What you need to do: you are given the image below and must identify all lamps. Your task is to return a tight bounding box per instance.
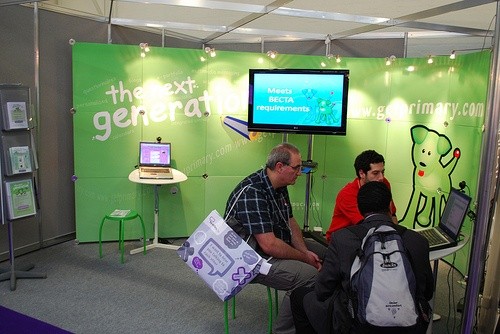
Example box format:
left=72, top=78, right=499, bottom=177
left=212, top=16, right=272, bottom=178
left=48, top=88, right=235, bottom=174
left=327, top=54, right=343, bottom=68
left=384, top=55, right=399, bottom=67
left=448, top=47, right=492, bottom=60
left=427, top=54, right=434, bottom=64
left=199, top=46, right=217, bottom=63
left=138, top=42, right=151, bottom=58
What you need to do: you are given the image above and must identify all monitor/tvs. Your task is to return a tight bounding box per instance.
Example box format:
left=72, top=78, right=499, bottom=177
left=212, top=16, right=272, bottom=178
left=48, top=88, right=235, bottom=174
left=248, top=69, right=349, bottom=135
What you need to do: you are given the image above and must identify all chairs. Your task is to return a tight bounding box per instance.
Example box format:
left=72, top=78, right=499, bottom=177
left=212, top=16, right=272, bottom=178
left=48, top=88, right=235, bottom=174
left=221, top=274, right=279, bottom=334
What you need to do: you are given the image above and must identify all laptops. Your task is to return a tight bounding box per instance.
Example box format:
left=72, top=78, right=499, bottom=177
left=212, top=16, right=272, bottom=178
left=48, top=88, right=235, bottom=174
left=416, top=187, right=472, bottom=251
left=138, top=142, right=172, bottom=179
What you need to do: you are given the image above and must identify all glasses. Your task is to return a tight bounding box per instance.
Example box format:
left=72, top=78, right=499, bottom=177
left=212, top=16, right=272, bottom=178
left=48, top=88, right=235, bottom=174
left=282, top=162, right=303, bottom=171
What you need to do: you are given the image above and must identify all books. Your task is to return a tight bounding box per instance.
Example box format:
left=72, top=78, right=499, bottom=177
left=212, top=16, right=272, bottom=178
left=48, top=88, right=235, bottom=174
left=109, top=209, right=130, bottom=218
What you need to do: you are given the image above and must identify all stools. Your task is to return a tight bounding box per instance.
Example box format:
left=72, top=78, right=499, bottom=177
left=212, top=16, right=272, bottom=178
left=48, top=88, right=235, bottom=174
left=98, top=209, right=148, bottom=265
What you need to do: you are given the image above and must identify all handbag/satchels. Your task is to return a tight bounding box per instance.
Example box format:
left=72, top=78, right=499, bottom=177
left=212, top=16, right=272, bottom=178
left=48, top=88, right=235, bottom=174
left=176, top=185, right=268, bottom=302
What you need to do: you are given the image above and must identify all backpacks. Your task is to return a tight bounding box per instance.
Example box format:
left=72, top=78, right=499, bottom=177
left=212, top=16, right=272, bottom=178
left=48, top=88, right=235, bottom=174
left=347, top=224, right=422, bottom=331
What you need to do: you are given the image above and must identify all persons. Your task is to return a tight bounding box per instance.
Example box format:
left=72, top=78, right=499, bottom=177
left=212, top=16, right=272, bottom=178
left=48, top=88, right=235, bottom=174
left=289, top=181, right=435, bottom=334
left=326, top=150, right=398, bottom=246
left=223, top=142, right=328, bottom=334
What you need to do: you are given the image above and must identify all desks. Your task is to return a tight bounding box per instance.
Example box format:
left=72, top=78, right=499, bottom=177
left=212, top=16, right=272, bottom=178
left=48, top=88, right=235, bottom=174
left=394, top=227, right=470, bottom=323
left=127, top=166, right=188, bottom=259
